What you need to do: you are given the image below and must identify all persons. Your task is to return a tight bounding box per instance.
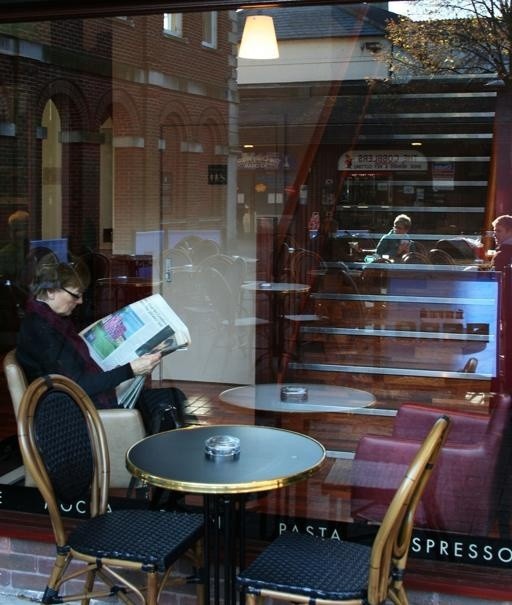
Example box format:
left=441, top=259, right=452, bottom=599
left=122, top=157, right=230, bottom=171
left=1, top=211, right=32, bottom=273
left=15, top=248, right=200, bottom=500
left=490, top=214, right=512, bottom=272
left=377, top=214, right=416, bottom=258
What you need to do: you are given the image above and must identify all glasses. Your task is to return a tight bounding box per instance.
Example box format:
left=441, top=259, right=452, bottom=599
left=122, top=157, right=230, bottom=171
left=60, top=286, right=82, bottom=302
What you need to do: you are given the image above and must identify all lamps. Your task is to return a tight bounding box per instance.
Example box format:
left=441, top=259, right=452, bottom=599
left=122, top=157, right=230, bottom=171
left=236, top=5, right=282, bottom=62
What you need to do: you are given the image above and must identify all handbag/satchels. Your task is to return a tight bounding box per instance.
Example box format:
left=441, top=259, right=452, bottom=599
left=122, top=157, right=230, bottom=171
left=136, top=382, right=186, bottom=435
